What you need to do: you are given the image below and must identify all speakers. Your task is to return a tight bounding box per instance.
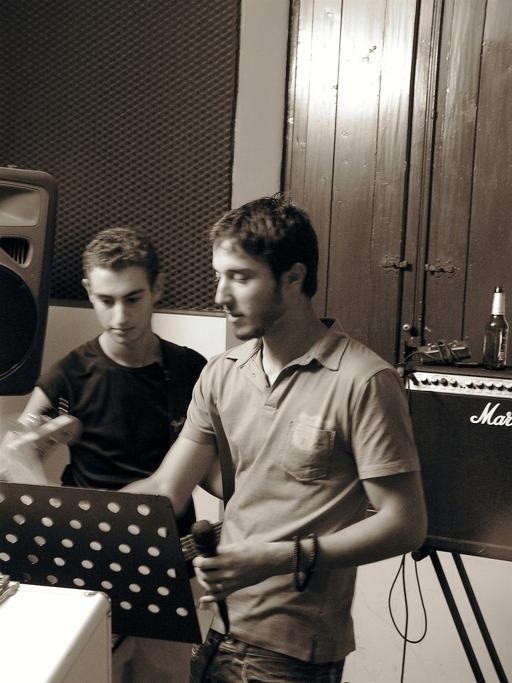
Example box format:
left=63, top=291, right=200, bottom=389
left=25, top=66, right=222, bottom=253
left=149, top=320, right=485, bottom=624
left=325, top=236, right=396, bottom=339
left=403, top=361, right=512, bottom=561
left=0, top=166, right=58, bottom=395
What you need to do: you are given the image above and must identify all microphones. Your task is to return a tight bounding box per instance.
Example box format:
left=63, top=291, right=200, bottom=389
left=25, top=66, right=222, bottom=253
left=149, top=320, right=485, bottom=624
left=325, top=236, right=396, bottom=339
left=193, top=519, right=229, bottom=637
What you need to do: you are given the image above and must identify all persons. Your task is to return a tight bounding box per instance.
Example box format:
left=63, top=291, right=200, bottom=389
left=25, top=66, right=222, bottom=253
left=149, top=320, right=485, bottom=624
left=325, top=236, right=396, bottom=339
left=119, top=199, right=427, bottom=683
left=1, top=228, right=223, bottom=683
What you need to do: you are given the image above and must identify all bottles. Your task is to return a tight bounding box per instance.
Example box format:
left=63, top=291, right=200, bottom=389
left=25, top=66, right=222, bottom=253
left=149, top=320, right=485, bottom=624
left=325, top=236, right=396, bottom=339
left=482, top=284, right=508, bottom=370
